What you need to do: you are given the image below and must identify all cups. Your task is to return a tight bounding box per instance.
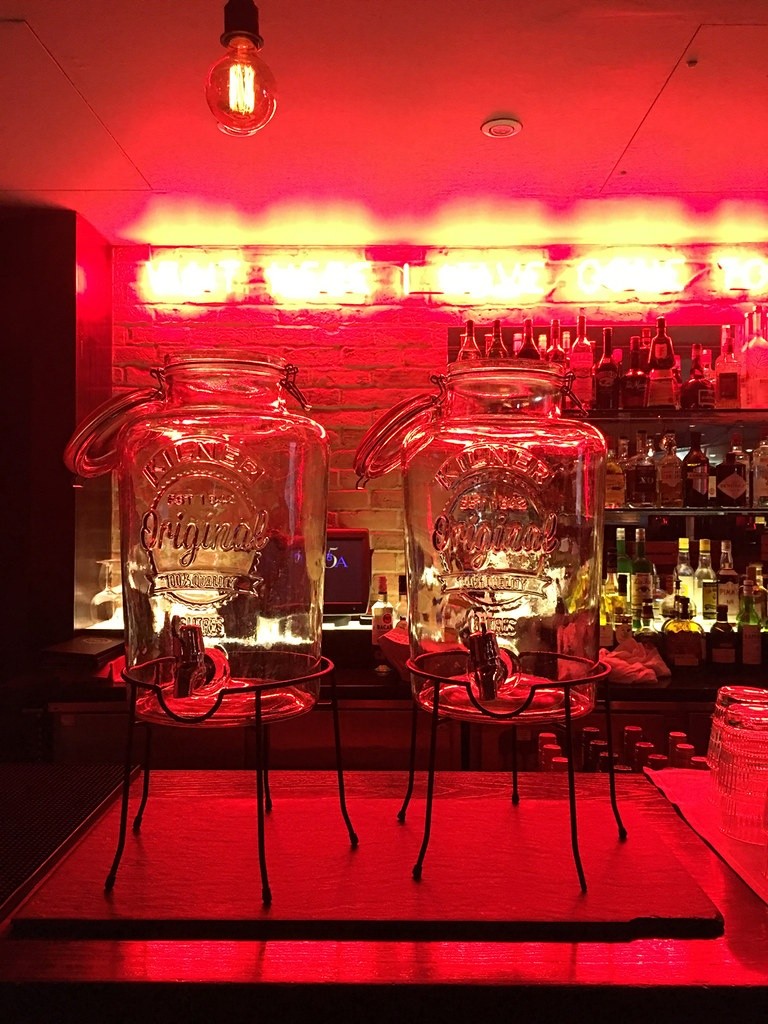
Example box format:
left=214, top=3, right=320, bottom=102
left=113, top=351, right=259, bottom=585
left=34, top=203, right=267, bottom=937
left=708, top=685, right=768, bottom=806
left=719, top=704, right=768, bottom=846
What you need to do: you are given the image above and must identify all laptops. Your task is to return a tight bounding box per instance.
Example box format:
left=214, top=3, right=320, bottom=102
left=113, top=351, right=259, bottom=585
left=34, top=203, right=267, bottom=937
left=262, top=528, right=371, bottom=621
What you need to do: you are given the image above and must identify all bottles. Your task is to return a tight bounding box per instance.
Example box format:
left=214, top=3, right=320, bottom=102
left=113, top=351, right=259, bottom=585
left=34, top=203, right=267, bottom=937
left=401, top=363, right=609, bottom=722
left=108, top=363, right=327, bottom=726
left=372, top=576, right=394, bottom=659
left=464, top=308, right=767, bottom=691
left=393, top=574, right=409, bottom=632
left=536, top=722, right=707, bottom=774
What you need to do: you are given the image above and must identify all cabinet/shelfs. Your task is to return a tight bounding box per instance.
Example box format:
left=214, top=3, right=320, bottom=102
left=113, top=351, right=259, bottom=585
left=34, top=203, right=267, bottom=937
left=559, top=410, right=768, bottom=700
